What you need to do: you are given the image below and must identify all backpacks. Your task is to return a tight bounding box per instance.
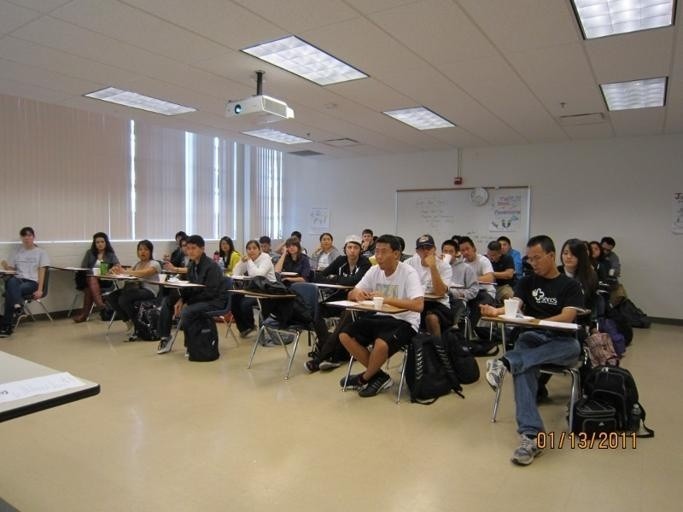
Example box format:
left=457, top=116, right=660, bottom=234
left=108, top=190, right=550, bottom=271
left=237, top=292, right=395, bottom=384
left=132, top=300, right=159, bottom=342
left=580, top=355, right=655, bottom=439
left=407, top=318, right=499, bottom=408
left=583, top=296, right=654, bottom=369
left=245, top=275, right=314, bottom=347
left=183, top=312, right=219, bottom=364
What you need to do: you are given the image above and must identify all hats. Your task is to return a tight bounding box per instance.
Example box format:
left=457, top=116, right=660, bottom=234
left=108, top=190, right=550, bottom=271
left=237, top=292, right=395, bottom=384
left=415, top=234, right=435, bottom=249
left=343, top=235, right=363, bottom=245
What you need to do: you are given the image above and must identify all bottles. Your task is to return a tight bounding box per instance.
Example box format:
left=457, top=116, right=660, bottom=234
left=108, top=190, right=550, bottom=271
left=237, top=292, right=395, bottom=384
left=213, top=250, right=224, bottom=269
left=100, top=261, right=107, bottom=276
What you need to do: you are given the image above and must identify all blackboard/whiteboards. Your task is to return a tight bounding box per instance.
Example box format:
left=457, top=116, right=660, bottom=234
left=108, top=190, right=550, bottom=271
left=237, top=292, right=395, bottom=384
left=396, top=184, right=531, bottom=257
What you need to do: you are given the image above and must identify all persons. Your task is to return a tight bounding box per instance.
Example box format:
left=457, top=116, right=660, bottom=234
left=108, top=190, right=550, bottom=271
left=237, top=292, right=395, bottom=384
left=478, top=234, right=620, bottom=466
left=109, top=230, right=339, bottom=359
left=72, top=231, right=121, bottom=323
left=304, top=229, right=524, bottom=397
left=0, top=226, right=50, bottom=337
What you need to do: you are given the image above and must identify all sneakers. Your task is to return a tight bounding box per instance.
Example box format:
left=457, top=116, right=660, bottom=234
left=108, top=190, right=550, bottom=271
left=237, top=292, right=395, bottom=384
left=484, top=357, right=508, bottom=392
left=240, top=325, right=258, bottom=339
left=0, top=328, right=13, bottom=337
left=304, top=358, right=342, bottom=373
left=15, top=306, right=28, bottom=320
left=510, top=434, right=545, bottom=467
left=358, top=369, right=393, bottom=397
left=156, top=335, right=173, bottom=355
left=340, top=372, right=368, bottom=390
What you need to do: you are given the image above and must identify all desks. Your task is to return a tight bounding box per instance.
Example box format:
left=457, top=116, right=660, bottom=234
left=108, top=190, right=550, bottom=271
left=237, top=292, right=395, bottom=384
left=44, top=263, right=619, bottom=441
left=0, top=349, right=101, bottom=512
left=0, top=269, right=18, bottom=279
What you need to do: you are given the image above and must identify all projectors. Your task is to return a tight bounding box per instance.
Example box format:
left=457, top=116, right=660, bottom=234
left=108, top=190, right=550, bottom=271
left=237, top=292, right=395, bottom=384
left=224, top=93, right=295, bottom=126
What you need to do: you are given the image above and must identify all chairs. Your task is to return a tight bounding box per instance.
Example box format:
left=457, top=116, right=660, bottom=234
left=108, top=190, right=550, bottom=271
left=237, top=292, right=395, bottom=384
left=10, top=267, right=55, bottom=334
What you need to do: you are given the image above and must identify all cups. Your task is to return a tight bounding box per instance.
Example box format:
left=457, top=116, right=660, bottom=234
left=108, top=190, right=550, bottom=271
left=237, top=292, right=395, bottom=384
left=92, top=268, right=100, bottom=276
left=502, top=298, right=519, bottom=318
left=373, top=297, right=384, bottom=309
left=442, top=253, right=452, bottom=264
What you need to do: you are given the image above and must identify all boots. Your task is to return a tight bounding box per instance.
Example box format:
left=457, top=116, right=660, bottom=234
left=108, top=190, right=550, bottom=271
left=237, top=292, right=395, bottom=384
left=72, top=304, right=93, bottom=323
left=92, top=293, right=106, bottom=309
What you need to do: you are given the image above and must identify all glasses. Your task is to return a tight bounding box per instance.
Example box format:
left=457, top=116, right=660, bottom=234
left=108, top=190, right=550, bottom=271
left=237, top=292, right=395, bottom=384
left=526, top=253, right=551, bottom=264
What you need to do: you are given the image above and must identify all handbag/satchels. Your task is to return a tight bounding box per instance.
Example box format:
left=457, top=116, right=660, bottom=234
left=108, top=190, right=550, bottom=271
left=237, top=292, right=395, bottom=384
left=567, top=397, right=619, bottom=441
